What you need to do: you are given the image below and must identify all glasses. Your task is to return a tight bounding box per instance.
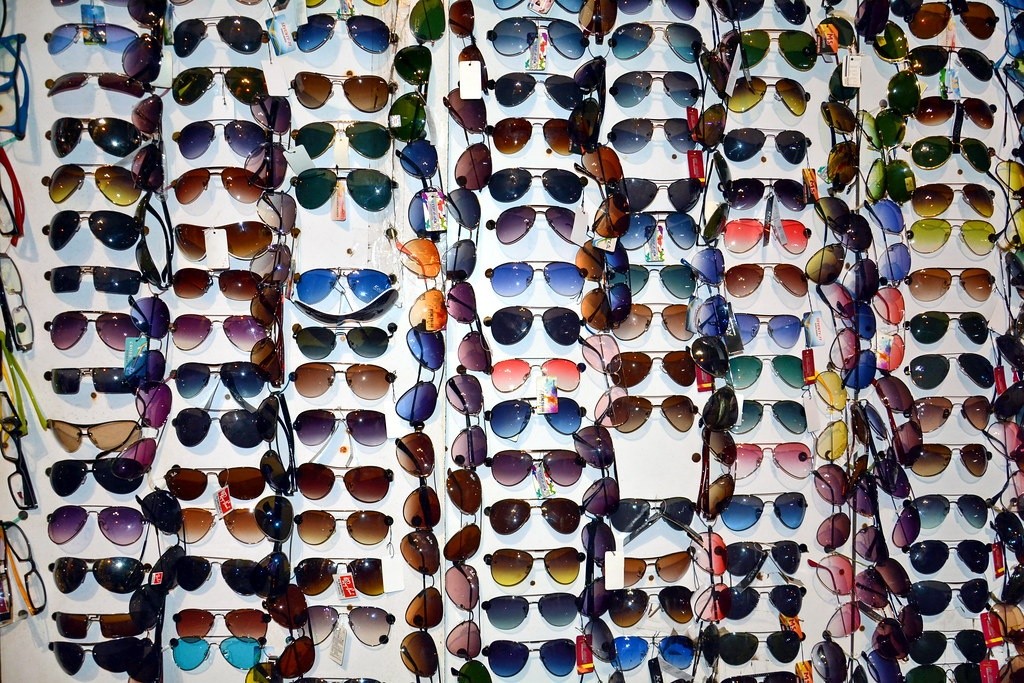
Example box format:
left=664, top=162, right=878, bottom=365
left=0, top=0, right=1024, bottom=683
